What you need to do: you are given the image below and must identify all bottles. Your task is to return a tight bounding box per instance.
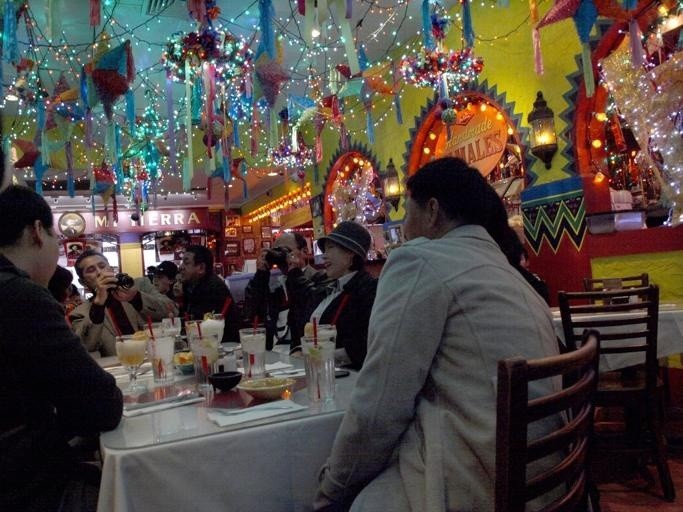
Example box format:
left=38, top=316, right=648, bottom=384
left=596, top=286, right=646, bottom=312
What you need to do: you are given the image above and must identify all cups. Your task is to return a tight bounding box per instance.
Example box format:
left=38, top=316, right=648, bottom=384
left=112, top=316, right=337, bottom=404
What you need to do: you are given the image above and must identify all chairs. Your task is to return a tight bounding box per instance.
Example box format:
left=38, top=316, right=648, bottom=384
left=582, top=273, right=649, bottom=304
left=490, top=328, right=601, bottom=511
left=557, top=284, right=675, bottom=503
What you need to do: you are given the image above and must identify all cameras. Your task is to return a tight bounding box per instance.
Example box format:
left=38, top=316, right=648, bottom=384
left=107, top=273, right=134, bottom=292
left=265, top=246, right=289, bottom=265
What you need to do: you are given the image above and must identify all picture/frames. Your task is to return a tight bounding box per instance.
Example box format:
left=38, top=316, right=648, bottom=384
left=243, top=238, right=256, bottom=254
left=226, top=215, right=240, bottom=228
left=224, top=240, right=240, bottom=256
left=242, top=225, right=253, bottom=234
left=261, top=226, right=272, bottom=239
left=261, top=241, right=271, bottom=249
left=225, top=228, right=237, bottom=237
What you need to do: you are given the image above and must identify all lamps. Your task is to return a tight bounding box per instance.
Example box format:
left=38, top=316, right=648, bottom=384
left=526, top=91, right=557, bottom=170
left=380, top=158, right=401, bottom=211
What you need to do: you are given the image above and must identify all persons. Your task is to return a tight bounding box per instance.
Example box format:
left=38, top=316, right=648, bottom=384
left=147, top=259, right=183, bottom=313
left=68, top=244, right=81, bottom=259
left=521, top=248, right=543, bottom=281
left=214, top=263, right=222, bottom=274
left=160, top=239, right=172, bottom=254
left=48, top=265, right=83, bottom=328
left=0, top=182, right=125, bottom=511
left=70, top=249, right=179, bottom=358
left=180, top=243, right=242, bottom=343
left=309, top=156, right=589, bottom=511
left=242, top=232, right=337, bottom=357
left=287, top=220, right=377, bottom=371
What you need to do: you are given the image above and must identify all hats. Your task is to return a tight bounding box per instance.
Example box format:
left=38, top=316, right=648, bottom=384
left=146, top=259, right=178, bottom=279
left=318, top=219, right=371, bottom=259
